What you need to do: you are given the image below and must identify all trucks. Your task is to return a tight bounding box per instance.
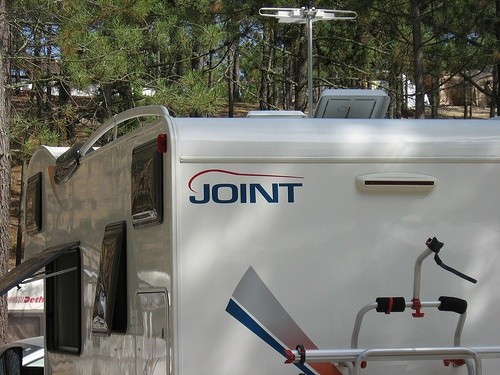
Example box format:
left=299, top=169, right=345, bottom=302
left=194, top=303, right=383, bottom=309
left=0, top=88, right=499, bottom=375
